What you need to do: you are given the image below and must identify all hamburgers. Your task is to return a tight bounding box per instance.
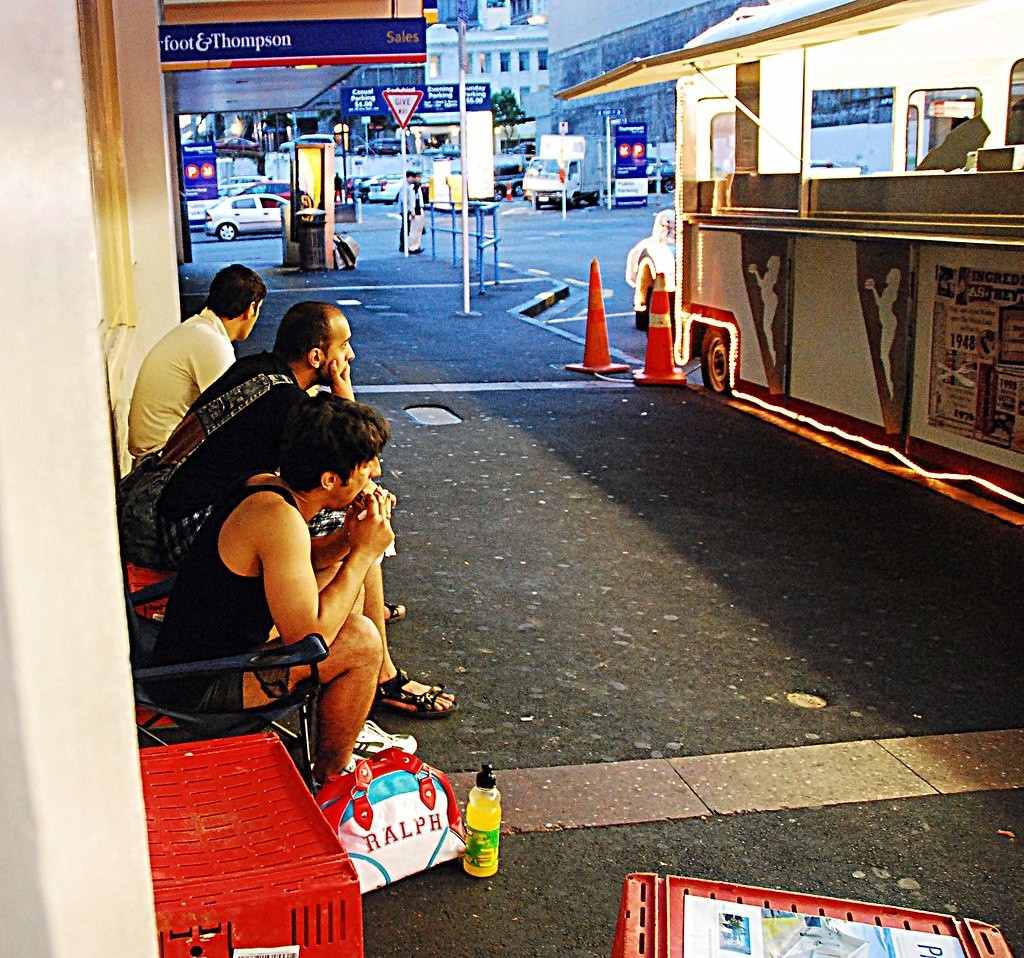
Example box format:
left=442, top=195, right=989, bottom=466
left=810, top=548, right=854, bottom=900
left=352, top=480, right=378, bottom=509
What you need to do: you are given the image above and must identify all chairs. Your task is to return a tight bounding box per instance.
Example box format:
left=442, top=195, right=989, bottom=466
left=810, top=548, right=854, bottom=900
left=125, top=565, right=327, bottom=786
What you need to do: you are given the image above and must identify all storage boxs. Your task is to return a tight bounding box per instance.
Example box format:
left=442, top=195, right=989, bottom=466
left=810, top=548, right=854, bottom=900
left=611, top=874, right=1014, bottom=958
left=138, top=730, right=363, bottom=958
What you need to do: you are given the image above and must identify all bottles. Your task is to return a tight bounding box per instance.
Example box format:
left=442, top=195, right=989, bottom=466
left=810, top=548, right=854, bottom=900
left=462, top=762, right=501, bottom=877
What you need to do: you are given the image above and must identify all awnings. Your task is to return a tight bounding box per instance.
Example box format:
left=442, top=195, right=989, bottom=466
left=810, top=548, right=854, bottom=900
left=162, top=65, right=361, bottom=113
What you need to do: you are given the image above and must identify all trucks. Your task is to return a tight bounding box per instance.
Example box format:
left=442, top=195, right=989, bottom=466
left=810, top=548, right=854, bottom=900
left=522, top=134, right=616, bottom=208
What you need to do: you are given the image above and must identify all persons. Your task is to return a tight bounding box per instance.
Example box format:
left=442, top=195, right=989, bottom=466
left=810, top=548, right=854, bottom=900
left=153, top=302, right=455, bottom=712
left=334, top=172, right=356, bottom=203
left=146, top=392, right=393, bottom=798
left=420, top=135, right=451, bottom=161
left=398, top=171, right=425, bottom=254
left=127, top=264, right=267, bottom=472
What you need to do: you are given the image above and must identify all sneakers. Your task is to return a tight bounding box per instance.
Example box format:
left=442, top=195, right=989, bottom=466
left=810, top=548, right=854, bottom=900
left=310, top=753, right=368, bottom=797
left=352, top=720, right=418, bottom=758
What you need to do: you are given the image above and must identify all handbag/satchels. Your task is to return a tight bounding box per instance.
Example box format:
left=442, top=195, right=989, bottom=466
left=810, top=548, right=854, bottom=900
left=316, top=747, right=467, bottom=895
left=118, top=454, right=170, bottom=567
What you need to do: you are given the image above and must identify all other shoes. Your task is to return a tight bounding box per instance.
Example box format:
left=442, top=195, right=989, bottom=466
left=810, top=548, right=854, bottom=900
left=409, top=247, right=425, bottom=255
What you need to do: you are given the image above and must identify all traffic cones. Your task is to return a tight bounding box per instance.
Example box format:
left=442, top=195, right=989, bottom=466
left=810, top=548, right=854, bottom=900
left=505, top=180, right=515, bottom=202
left=632, top=272, right=689, bottom=386
left=566, top=256, right=631, bottom=372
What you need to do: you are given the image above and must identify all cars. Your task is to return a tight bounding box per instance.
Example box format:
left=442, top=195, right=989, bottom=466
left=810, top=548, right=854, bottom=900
left=646, top=156, right=676, bottom=194
left=501, top=140, right=537, bottom=155
left=355, top=173, right=429, bottom=207
left=204, top=194, right=291, bottom=240
left=215, top=137, right=263, bottom=157
left=234, top=180, right=291, bottom=207
left=420, top=142, right=462, bottom=159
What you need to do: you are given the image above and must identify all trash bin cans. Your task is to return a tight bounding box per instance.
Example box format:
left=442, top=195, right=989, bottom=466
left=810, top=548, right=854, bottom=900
left=296, top=207, right=327, bottom=271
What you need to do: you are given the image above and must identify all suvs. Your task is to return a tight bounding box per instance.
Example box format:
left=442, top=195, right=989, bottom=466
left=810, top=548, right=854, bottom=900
left=217, top=175, right=269, bottom=198
left=278, top=133, right=345, bottom=156
left=354, top=137, right=411, bottom=156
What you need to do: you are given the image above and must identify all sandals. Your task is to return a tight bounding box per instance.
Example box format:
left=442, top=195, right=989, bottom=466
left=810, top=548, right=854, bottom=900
left=375, top=668, right=460, bottom=718
left=384, top=602, right=406, bottom=622
